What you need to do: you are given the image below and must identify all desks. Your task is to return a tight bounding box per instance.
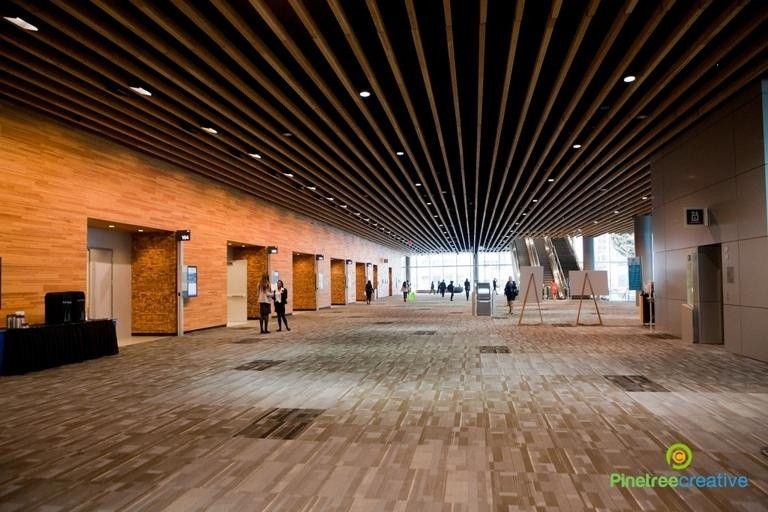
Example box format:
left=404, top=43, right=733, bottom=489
left=0, top=320, right=119, bottom=376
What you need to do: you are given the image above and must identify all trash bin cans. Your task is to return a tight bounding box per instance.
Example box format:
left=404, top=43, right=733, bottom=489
left=476, top=282, right=496, bottom=317
left=639, top=293, right=656, bottom=326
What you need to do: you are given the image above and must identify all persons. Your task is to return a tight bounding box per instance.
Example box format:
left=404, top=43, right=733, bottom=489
left=271, top=279, right=290, bottom=332
left=492, top=278, right=499, bottom=295
left=438, top=279, right=447, bottom=297
left=550, top=279, right=557, bottom=300
left=255, top=272, right=276, bottom=333
left=365, top=281, right=373, bottom=304
left=437, top=281, right=441, bottom=294
left=448, top=281, right=455, bottom=301
left=428, top=281, right=436, bottom=295
left=464, top=278, right=471, bottom=300
left=401, top=281, right=409, bottom=301
left=504, top=276, right=518, bottom=313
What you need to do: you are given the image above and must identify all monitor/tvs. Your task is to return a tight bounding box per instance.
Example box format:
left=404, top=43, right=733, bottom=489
left=478, top=288, right=490, bottom=294
